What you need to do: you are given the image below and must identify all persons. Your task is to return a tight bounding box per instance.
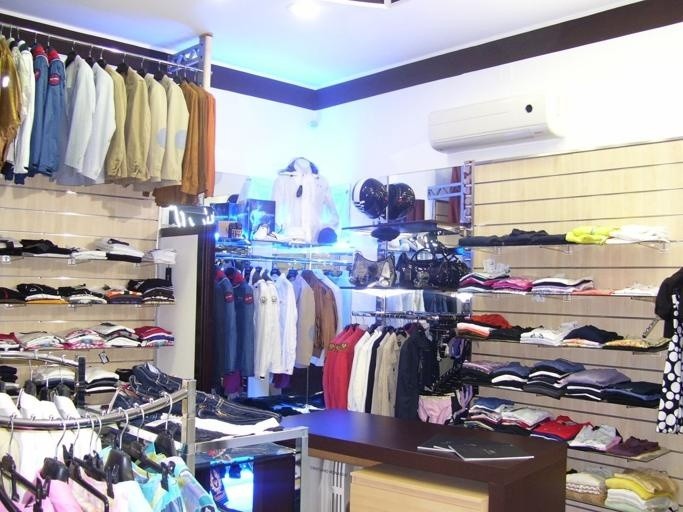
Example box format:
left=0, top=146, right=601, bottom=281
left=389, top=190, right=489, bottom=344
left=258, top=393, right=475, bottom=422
left=248, top=201, right=274, bottom=240
left=272, top=157, right=338, bottom=243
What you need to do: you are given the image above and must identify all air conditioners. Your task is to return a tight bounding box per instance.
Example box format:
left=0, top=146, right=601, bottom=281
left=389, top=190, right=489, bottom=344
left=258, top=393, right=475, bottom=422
left=427, top=92, right=566, bottom=154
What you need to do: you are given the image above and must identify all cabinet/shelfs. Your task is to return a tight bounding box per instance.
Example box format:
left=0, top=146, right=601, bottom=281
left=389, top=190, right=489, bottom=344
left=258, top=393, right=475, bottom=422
left=457, top=238, right=672, bottom=511
left=339, top=220, right=461, bottom=292
left=273, top=409, right=569, bottom=511
left=0, top=252, right=175, bottom=350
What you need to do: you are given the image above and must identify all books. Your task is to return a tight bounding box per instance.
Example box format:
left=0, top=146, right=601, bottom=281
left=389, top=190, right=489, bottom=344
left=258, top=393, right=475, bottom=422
left=416, top=438, right=533, bottom=463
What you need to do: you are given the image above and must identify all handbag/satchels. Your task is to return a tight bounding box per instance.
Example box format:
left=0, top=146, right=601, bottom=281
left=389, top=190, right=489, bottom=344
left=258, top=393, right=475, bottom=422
left=349, top=247, right=471, bottom=289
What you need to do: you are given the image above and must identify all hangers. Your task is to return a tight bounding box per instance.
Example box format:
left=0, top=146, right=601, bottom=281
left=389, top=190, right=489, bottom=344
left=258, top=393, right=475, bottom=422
left=0, top=393, right=177, bottom=512
left=345, top=312, right=420, bottom=337
left=1, top=24, right=198, bottom=85
left=217, top=252, right=324, bottom=281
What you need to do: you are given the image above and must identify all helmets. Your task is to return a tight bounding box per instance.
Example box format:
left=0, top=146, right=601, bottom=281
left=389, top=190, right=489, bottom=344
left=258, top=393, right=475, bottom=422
left=388, top=183, right=415, bottom=220
left=352, top=178, right=387, bottom=218
